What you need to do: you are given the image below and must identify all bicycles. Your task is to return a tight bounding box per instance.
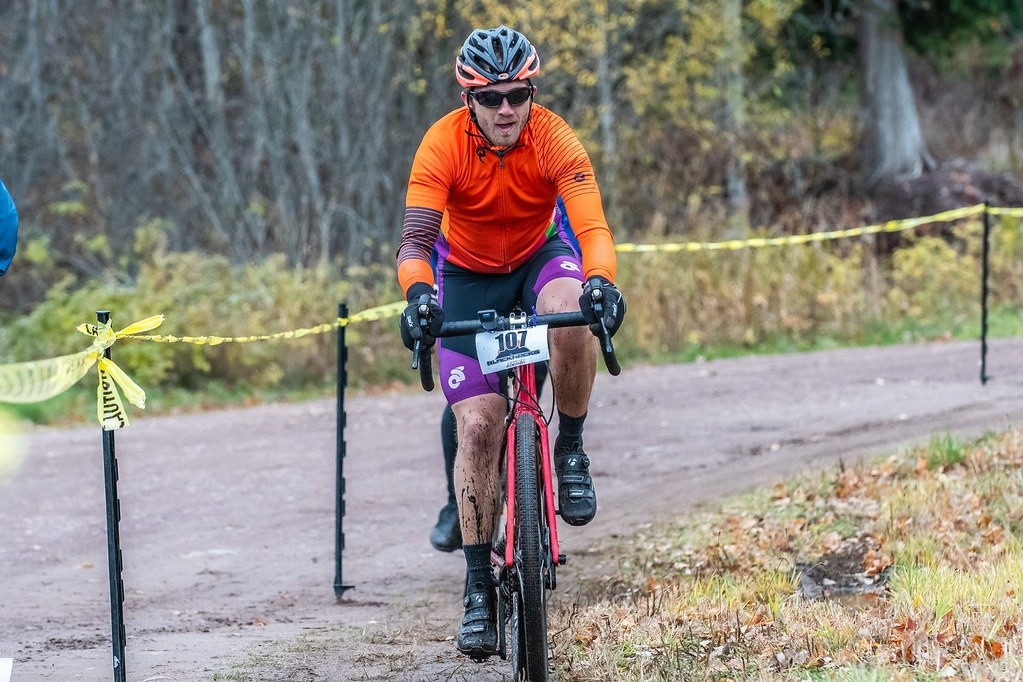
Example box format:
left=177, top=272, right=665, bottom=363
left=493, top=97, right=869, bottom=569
left=411, top=278, right=621, bottom=681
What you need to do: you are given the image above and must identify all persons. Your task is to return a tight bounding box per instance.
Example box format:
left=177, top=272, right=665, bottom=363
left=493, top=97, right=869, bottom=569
left=394, top=25, right=626, bottom=654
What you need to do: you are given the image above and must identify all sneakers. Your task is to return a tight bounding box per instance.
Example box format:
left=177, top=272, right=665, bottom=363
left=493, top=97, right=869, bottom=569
left=456, top=572, right=499, bottom=657
left=430, top=496, right=462, bottom=552
left=552, top=436, right=598, bottom=526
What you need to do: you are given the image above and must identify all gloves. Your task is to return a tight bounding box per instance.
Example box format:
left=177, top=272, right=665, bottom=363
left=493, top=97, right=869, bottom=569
left=580, top=275, right=629, bottom=339
left=397, top=293, right=446, bottom=354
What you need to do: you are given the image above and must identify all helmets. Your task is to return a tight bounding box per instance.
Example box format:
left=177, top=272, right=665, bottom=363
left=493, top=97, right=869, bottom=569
left=455, top=24, right=540, bottom=87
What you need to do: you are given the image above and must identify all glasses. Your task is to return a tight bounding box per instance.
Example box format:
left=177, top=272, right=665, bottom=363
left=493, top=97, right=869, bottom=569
left=469, top=86, right=534, bottom=107
